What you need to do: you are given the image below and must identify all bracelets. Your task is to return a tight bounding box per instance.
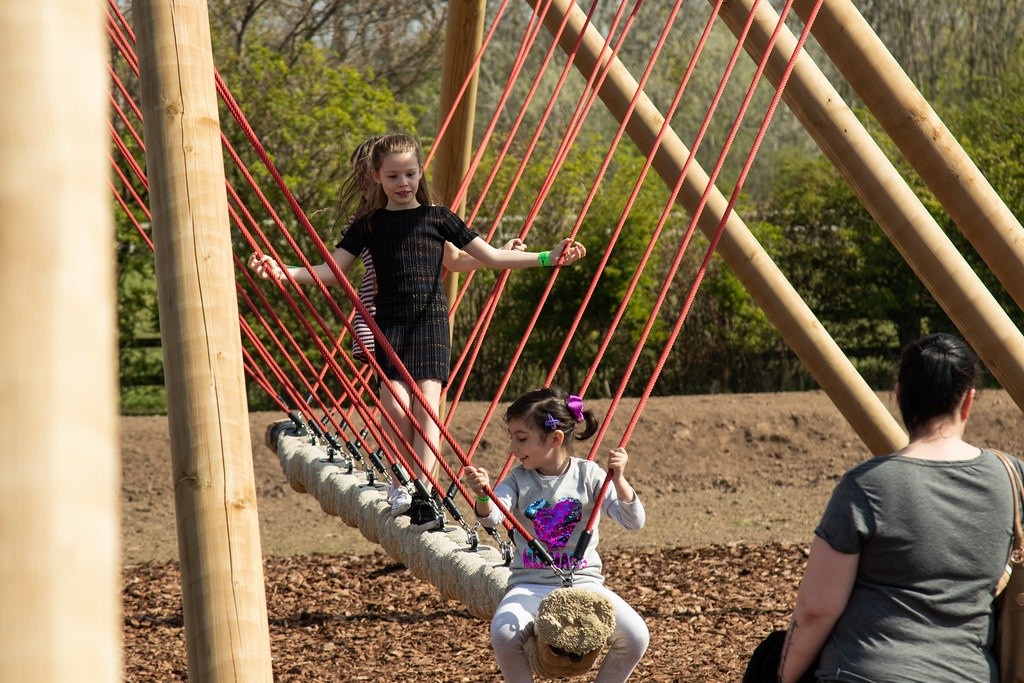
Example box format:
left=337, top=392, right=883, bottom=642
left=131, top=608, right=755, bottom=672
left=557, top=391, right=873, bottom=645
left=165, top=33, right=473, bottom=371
left=539, top=251, right=551, bottom=266
left=477, top=496, right=489, bottom=502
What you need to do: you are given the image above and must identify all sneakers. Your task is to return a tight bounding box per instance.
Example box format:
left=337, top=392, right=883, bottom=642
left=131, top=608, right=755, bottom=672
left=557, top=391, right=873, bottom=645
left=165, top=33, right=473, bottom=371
left=387, top=484, right=413, bottom=516
left=409, top=490, right=442, bottom=532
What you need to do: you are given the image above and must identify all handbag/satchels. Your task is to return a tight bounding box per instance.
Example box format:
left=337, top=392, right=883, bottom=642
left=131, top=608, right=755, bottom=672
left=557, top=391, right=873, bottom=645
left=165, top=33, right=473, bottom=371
left=987, top=446, right=1024, bottom=683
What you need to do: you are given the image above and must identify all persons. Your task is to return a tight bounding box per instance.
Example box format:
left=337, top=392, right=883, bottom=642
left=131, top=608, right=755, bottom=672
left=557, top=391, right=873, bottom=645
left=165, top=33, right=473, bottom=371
left=263, top=136, right=527, bottom=516
left=249, top=134, right=587, bottom=531
left=465, top=389, right=649, bottom=683
left=778, top=334, right=1024, bottom=683
